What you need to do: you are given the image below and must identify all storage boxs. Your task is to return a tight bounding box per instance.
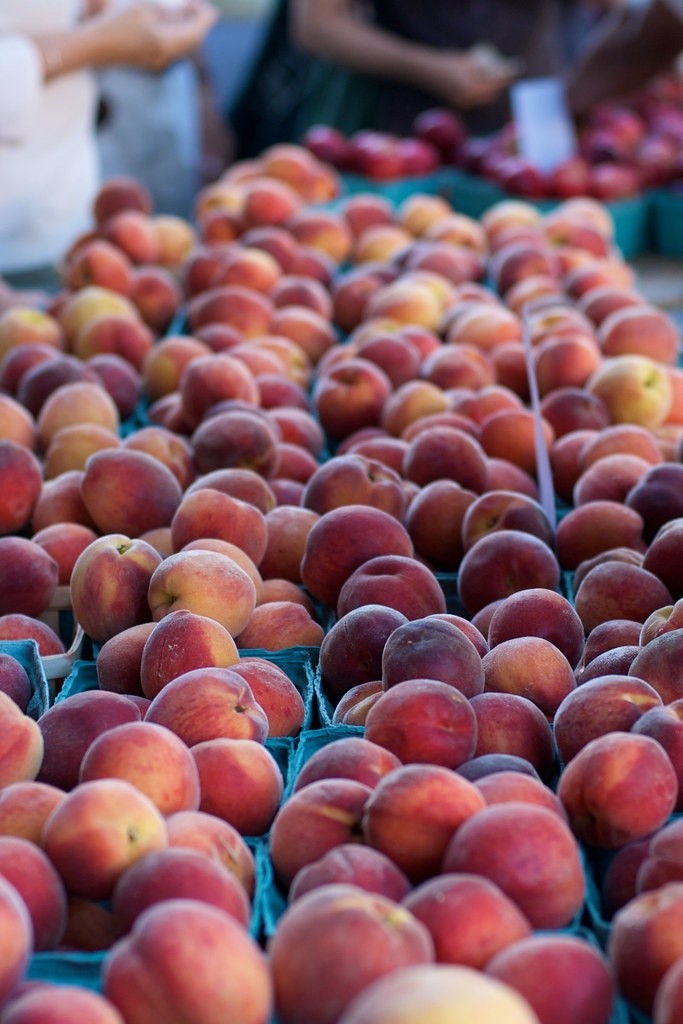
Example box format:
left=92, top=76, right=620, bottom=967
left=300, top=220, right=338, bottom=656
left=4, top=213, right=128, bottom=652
left=0, top=168, right=683, bottom=1024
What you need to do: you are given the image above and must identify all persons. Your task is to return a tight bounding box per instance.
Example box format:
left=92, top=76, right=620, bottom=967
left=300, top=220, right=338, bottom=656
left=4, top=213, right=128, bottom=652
left=228, top=0, right=543, bottom=163
left=0, top=0, right=219, bottom=291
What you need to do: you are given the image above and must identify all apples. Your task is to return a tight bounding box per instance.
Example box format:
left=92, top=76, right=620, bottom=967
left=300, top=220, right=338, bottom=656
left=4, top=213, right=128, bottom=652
left=301, top=70, right=682, bottom=197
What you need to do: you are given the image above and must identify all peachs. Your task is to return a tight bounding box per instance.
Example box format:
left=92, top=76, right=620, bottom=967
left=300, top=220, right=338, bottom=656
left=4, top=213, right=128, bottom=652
left=0, top=142, right=683, bottom=1024
